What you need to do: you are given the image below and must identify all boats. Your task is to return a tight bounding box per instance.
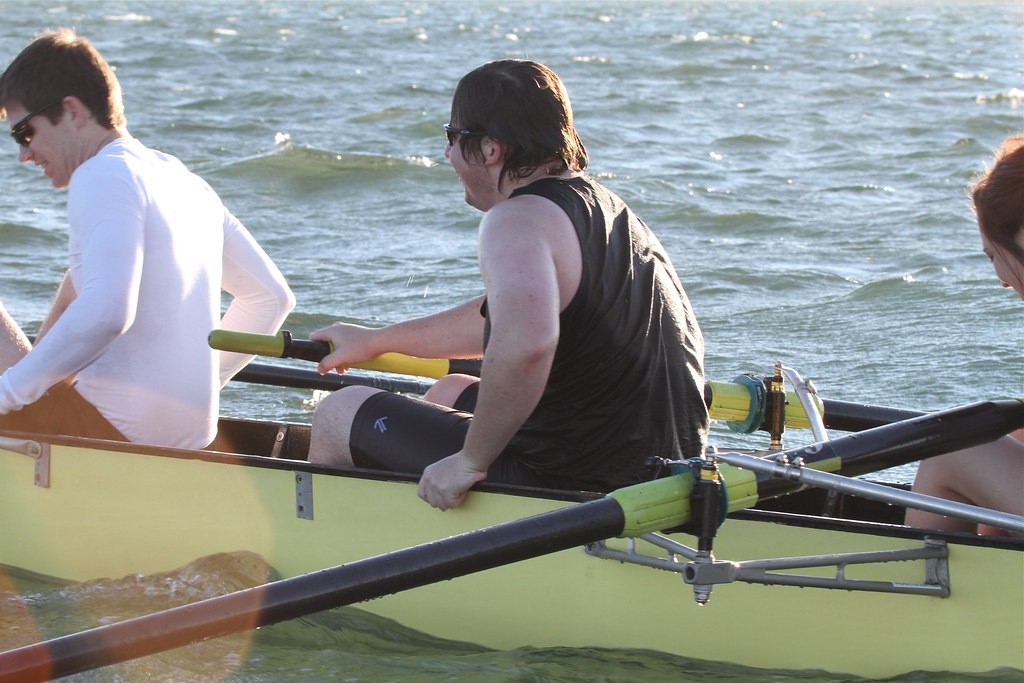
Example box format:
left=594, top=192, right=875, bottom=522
left=0, top=416, right=1023, bottom=683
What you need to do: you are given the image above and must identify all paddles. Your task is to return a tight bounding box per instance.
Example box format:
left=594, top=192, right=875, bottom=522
left=0, top=398, right=1022, bottom=682
left=206, top=327, right=923, bottom=430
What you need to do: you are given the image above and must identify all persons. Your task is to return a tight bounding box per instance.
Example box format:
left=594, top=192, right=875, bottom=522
left=0, top=27, right=298, bottom=451
left=903, top=144, right=1024, bottom=539
left=306, top=59, right=711, bottom=512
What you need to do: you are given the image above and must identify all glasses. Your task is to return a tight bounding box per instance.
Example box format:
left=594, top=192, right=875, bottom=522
left=443, top=123, right=486, bottom=146
left=9, top=91, right=89, bottom=148
